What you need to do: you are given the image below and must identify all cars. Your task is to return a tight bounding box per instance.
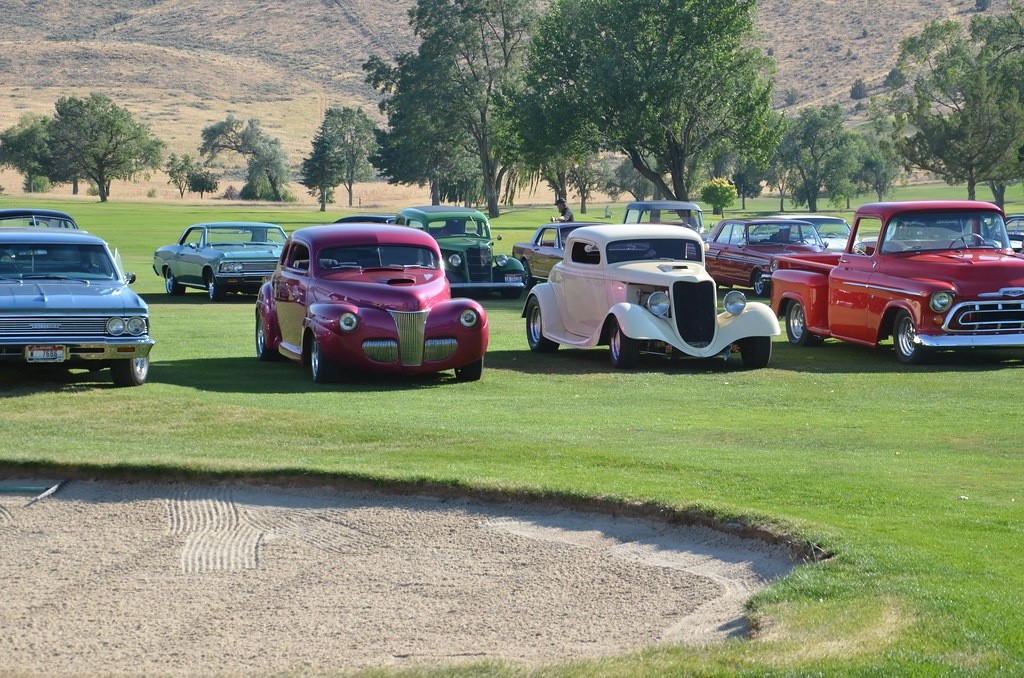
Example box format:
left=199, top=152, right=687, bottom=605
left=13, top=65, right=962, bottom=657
left=255, top=223, right=488, bottom=383
left=0, top=227, right=156, bottom=385
left=0, top=201, right=1024, bottom=302
left=521, top=224, right=782, bottom=369
left=770, top=201, right=1024, bottom=363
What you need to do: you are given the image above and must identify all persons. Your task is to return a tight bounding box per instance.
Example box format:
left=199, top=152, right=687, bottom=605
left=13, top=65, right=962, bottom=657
left=550, top=197, right=574, bottom=222
left=605, top=205, right=612, bottom=219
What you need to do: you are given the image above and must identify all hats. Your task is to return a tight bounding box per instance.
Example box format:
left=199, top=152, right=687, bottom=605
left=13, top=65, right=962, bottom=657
left=554, top=198, right=565, bottom=205
